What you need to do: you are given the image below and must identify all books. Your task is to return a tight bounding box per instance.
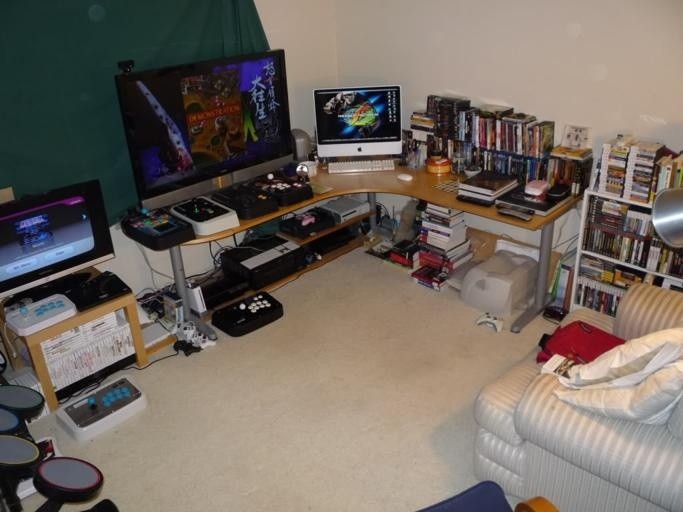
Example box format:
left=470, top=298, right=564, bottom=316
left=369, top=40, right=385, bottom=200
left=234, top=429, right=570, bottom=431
left=362, top=201, right=477, bottom=293
left=404, top=93, right=594, bottom=223
left=548, top=133, right=682, bottom=317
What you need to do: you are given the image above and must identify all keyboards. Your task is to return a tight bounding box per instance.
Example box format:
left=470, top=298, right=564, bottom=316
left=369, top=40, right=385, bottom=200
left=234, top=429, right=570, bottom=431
left=327, top=160, right=395, bottom=174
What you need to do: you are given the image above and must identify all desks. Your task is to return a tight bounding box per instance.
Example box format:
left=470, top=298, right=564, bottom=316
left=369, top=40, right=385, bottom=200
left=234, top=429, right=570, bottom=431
left=0, top=259, right=150, bottom=412
left=168, top=156, right=585, bottom=337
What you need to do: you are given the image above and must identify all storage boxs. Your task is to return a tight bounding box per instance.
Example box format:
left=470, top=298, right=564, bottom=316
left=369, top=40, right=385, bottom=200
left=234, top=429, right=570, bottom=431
left=455, top=248, right=540, bottom=319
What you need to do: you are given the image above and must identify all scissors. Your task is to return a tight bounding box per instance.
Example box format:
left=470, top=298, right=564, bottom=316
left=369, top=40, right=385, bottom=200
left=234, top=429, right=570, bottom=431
left=411, top=139, right=421, bottom=163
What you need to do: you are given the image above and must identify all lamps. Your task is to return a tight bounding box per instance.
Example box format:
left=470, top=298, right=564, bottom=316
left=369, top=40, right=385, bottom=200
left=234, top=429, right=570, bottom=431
left=648, top=186, right=682, bottom=250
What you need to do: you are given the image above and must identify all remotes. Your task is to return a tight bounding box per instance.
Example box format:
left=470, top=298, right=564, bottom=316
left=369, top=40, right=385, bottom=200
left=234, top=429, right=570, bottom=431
left=456, top=195, right=492, bottom=207
left=497, top=208, right=532, bottom=222
left=495, top=201, right=535, bottom=215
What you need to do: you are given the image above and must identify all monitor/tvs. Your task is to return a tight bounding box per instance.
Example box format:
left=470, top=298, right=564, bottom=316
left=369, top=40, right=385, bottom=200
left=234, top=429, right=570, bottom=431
left=0, top=178, right=115, bottom=310
left=114, top=49, right=294, bottom=214
left=312, top=85, right=402, bottom=157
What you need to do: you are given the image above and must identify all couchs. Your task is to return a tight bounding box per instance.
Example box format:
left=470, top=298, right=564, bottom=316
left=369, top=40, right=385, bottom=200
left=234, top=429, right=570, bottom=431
left=467, top=279, right=682, bottom=512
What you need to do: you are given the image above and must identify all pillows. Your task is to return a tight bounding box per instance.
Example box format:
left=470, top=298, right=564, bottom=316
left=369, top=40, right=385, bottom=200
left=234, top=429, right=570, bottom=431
left=556, top=326, right=681, bottom=390
left=551, top=360, right=682, bottom=425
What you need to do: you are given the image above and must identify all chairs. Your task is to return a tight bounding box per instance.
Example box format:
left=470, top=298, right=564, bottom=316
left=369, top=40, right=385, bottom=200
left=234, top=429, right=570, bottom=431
left=409, top=477, right=563, bottom=511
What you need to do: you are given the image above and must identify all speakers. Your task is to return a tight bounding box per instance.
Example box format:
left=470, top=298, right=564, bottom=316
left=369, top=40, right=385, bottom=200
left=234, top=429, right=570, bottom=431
left=290, top=129, right=312, bottom=162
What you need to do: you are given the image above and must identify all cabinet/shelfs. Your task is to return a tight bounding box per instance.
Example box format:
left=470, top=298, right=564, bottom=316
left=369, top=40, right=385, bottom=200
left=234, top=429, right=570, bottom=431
left=564, top=184, right=683, bottom=319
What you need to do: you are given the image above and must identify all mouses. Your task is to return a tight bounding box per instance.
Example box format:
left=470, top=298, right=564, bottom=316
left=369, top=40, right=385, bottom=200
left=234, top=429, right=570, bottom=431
left=396, top=172, right=412, bottom=181
